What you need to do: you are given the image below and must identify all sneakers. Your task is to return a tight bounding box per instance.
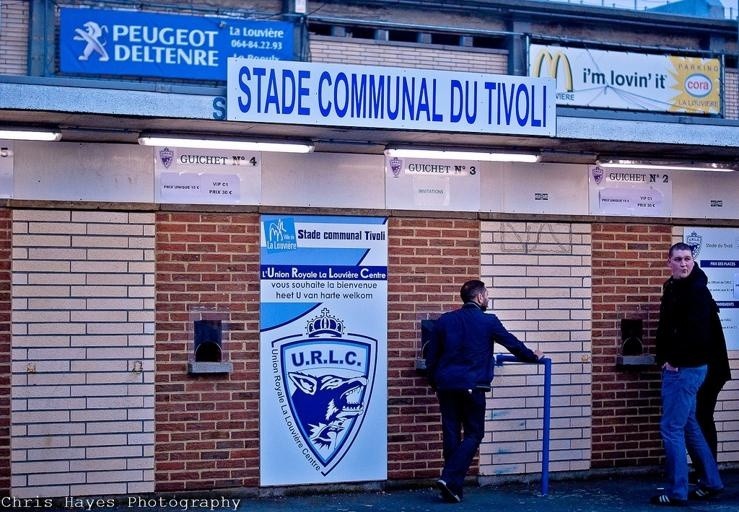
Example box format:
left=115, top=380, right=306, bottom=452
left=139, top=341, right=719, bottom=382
left=649, top=483, right=724, bottom=506
left=436, top=480, right=460, bottom=502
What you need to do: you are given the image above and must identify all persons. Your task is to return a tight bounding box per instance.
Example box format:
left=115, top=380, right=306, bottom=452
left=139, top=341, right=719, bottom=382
left=688, top=286, right=731, bottom=485
left=427, top=280, right=545, bottom=503
left=651, top=243, right=724, bottom=507
